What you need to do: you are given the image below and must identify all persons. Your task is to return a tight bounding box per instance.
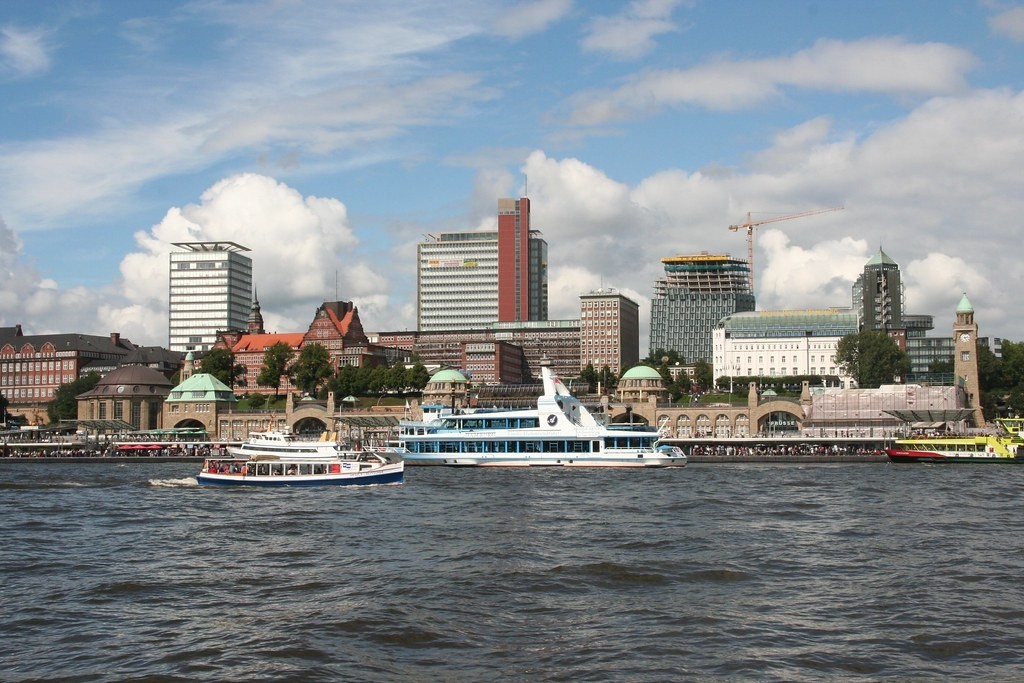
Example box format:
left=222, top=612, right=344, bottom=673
left=693, top=390, right=710, bottom=404
left=1, top=426, right=328, bottom=474
left=659, top=416, right=1022, bottom=457
left=453, top=403, right=533, bottom=430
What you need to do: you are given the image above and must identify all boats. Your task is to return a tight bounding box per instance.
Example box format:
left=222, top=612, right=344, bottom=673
left=225, top=431, right=338, bottom=458
left=386, top=364, right=687, bottom=469
left=883, top=418, right=1024, bottom=463
left=196, top=450, right=405, bottom=488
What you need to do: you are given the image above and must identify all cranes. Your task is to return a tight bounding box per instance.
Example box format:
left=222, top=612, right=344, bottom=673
left=728, top=205, right=845, bottom=299
